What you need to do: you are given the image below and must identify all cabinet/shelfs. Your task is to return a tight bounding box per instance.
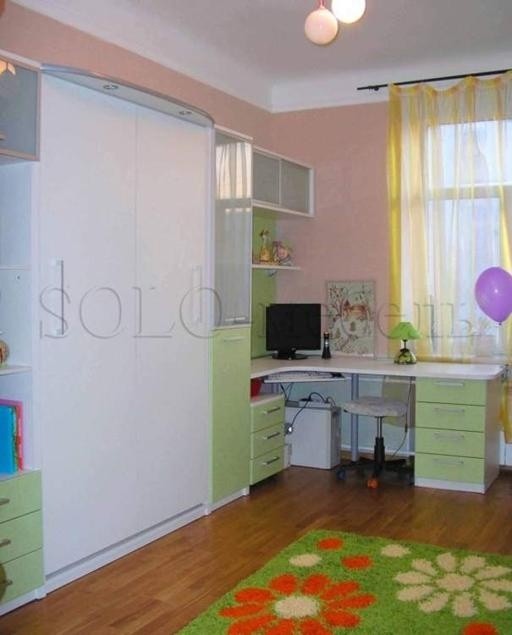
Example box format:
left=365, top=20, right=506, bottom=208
left=0, top=466, right=45, bottom=605
left=212, top=124, right=253, bottom=331
left=0, top=48, right=211, bottom=602
left=211, top=323, right=252, bottom=504
left=250, top=392, right=285, bottom=486
left=414, top=377, right=501, bottom=495
left=252, top=144, right=314, bottom=218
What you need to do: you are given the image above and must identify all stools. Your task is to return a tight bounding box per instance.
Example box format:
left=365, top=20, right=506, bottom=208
left=336, top=396, right=408, bottom=489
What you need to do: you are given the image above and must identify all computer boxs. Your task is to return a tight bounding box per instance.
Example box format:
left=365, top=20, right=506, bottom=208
left=284, top=400, right=342, bottom=470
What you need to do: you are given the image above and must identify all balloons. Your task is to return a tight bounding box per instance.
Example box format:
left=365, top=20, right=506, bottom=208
left=473, top=266, right=512, bottom=325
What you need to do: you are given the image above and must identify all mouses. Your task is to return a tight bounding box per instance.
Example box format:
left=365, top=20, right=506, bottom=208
left=332, top=373, right=341, bottom=377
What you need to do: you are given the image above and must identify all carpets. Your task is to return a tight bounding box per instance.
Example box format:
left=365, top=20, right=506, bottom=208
left=171, top=528, right=512, bottom=635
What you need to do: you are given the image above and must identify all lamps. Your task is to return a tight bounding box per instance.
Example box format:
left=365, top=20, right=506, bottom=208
left=387, top=321, right=421, bottom=365
left=303, top=0, right=338, bottom=46
left=331, top=0, right=366, bottom=25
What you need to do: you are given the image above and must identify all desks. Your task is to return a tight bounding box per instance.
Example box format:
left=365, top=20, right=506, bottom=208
left=251, top=353, right=507, bottom=464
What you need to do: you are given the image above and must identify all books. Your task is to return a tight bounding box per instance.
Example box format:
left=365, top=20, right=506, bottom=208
left=0, top=398, right=25, bottom=474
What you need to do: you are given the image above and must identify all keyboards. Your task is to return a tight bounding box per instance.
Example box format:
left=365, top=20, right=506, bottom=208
left=268, top=371, right=335, bottom=380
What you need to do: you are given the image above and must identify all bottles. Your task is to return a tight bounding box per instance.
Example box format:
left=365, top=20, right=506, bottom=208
left=322, top=331, right=331, bottom=358
left=259, top=230, right=271, bottom=263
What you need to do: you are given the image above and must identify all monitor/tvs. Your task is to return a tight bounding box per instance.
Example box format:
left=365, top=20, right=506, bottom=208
left=268, top=303, right=321, bottom=360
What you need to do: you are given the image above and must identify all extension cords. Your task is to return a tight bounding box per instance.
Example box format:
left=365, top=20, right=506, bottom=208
left=299, top=398, right=332, bottom=408
